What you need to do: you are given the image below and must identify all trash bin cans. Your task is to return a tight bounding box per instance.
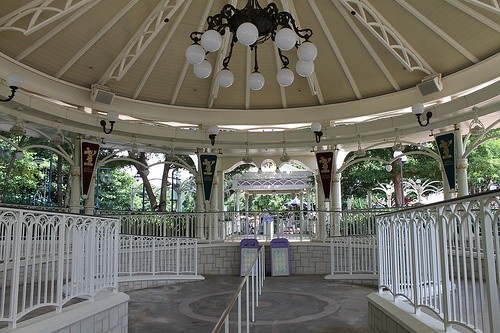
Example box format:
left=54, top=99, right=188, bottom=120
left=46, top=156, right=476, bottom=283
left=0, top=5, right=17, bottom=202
left=269, top=237, right=291, bottom=277
left=239, top=238, right=259, bottom=277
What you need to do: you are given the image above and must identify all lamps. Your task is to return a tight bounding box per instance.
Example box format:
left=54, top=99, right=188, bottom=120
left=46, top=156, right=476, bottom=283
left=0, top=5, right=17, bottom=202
left=311, top=121, right=323, bottom=143
left=207, top=125, right=219, bottom=146
left=100, top=110, right=119, bottom=134
left=0, top=72, right=24, bottom=102
left=411, top=102, right=432, bottom=126
left=185, top=0, right=318, bottom=91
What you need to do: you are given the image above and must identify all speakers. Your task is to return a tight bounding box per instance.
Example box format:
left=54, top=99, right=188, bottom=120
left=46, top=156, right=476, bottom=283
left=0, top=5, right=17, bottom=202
left=90, top=89, right=115, bottom=105
left=417, top=77, right=443, bottom=96
